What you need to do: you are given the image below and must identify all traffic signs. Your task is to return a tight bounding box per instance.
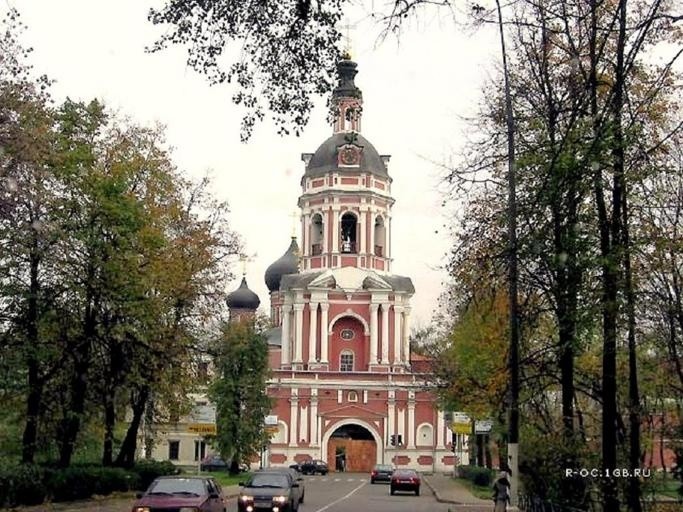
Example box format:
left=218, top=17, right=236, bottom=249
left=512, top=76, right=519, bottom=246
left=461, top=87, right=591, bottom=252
left=187, top=424, right=216, bottom=433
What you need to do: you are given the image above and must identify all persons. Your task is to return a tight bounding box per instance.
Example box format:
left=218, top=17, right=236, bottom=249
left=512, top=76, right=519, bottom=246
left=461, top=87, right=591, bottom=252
left=492, top=471, right=510, bottom=512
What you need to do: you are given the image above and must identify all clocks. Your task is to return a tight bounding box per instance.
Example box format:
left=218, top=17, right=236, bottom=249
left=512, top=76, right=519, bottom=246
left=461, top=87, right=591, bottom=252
left=340, top=149, right=358, bottom=166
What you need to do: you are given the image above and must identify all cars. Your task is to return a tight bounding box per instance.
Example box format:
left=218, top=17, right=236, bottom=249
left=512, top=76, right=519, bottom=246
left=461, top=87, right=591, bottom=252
left=132, top=476, right=227, bottom=512
left=371, top=464, right=396, bottom=484
left=237, top=466, right=305, bottom=512
left=201, top=454, right=250, bottom=472
left=391, top=468, right=420, bottom=496
left=289, top=459, right=329, bottom=475
